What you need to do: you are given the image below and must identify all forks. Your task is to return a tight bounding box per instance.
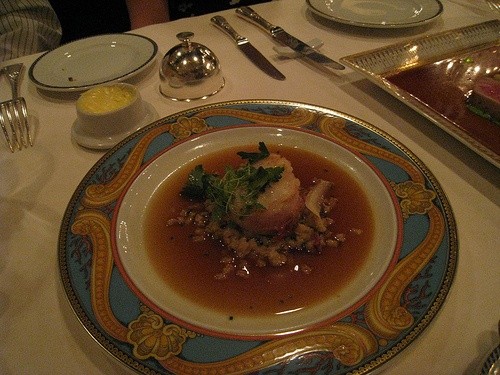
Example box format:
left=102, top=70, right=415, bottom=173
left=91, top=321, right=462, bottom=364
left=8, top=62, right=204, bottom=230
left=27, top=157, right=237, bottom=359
left=486, top=0, right=500, bottom=11
left=0, top=63, right=32, bottom=153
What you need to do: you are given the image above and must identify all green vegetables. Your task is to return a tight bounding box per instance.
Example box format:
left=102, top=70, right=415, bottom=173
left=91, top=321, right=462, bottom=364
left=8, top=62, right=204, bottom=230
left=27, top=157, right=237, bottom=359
left=466, top=102, right=500, bottom=129
left=177, top=141, right=319, bottom=255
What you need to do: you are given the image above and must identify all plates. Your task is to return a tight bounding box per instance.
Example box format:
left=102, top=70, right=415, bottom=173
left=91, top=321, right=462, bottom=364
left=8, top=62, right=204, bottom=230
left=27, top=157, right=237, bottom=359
left=305, top=0, right=444, bottom=29
left=28, top=32, right=159, bottom=92
left=57, top=98, right=459, bottom=375
left=338, top=20, right=500, bottom=168
left=476, top=342, right=500, bottom=375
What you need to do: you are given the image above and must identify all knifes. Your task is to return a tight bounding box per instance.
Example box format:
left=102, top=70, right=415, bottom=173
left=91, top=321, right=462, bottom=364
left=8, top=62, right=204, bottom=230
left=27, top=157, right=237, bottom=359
left=210, top=15, right=287, bottom=81
left=235, top=6, right=346, bottom=70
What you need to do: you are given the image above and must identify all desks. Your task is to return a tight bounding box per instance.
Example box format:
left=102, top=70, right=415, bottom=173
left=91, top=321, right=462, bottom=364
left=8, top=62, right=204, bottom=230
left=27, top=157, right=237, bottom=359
left=0, top=0, right=500, bottom=375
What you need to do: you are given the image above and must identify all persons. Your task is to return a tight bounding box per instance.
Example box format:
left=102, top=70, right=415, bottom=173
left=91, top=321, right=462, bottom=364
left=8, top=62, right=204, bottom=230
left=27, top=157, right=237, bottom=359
left=0, top=0, right=278, bottom=63
